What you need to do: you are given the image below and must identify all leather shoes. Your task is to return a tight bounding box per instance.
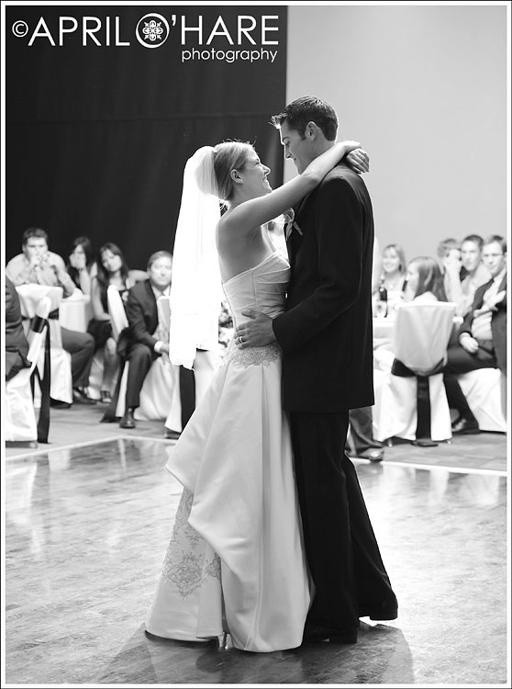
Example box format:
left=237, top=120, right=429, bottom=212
left=357, top=605, right=396, bottom=621
left=303, top=621, right=357, bottom=643
left=450, top=417, right=479, bottom=434
left=120, top=414, right=135, bottom=428
left=73, top=387, right=113, bottom=404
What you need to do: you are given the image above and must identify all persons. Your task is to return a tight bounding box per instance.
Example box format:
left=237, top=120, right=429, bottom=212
left=145, top=140, right=369, bottom=653
left=233, top=96, right=398, bottom=650
left=7, top=227, right=506, bottom=461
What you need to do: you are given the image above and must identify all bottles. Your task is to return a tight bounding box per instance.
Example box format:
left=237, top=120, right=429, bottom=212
left=379, top=279, right=387, bottom=318
